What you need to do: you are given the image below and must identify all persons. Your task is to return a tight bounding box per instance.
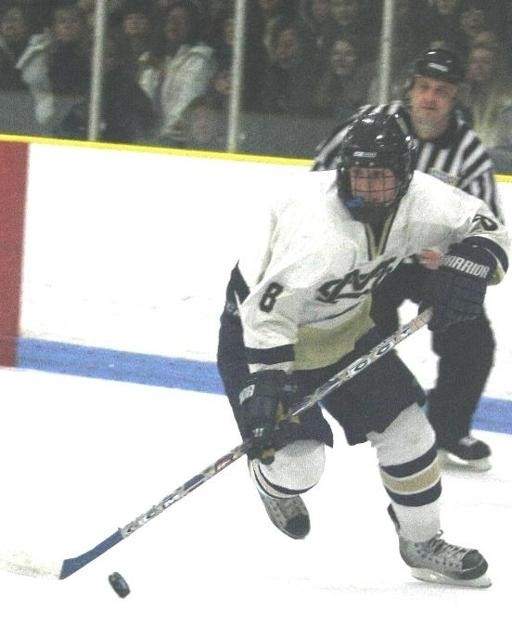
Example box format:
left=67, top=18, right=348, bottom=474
left=304, top=46, right=497, bottom=463
left=0, top=1, right=509, bottom=177
left=215, top=112, right=512, bottom=581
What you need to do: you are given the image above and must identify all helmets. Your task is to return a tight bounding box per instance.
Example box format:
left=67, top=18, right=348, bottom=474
left=411, top=47, right=465, bottom=86
left=335, top=113, right=417, bottom=223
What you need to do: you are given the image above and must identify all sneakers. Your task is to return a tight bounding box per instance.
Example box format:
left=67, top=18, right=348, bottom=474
left=436, top=435, right=492, bottom=461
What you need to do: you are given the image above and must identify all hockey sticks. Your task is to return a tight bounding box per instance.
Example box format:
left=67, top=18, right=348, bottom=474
left=3, top=305, right=433, bottom=577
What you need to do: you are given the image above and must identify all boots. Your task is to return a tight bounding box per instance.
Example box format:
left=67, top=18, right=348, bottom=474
left=248, top=458, right=311, bottom=539
left=386, top=504, right=490, bottom=580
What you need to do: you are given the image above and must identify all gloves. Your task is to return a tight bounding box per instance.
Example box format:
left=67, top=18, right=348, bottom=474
left=418, top=242, right=495, bottom=334
left=237, top=369, right=300, bottom=464
